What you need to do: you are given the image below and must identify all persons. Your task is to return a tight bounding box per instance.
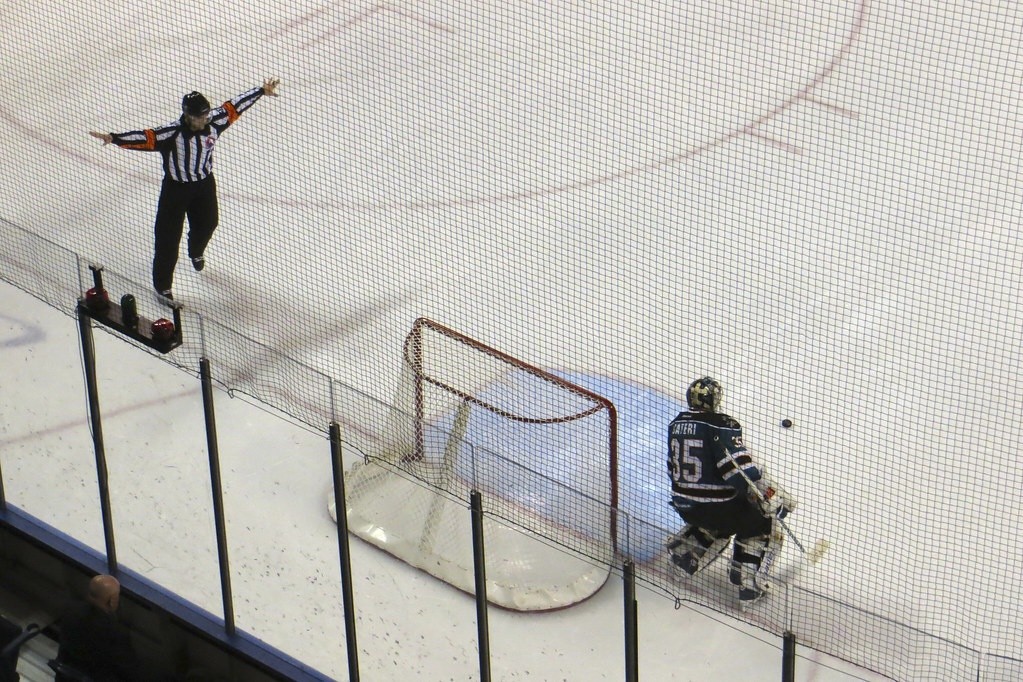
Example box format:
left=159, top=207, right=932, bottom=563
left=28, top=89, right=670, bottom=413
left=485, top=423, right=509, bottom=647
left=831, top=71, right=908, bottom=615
left=667, top=377, right=797, bottom=611
left=90, top=78, right=278, bottom=304
left=57, top=575, right=152, bottom=682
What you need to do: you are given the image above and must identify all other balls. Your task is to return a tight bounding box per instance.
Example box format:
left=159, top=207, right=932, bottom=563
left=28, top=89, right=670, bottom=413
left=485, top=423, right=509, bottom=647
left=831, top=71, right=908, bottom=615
left=782, top=419, right=792, bottom=428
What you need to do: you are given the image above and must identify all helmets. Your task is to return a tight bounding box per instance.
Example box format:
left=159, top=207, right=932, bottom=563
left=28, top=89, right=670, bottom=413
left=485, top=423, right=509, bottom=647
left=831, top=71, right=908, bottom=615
left=686, top=375, right=726, bottom=414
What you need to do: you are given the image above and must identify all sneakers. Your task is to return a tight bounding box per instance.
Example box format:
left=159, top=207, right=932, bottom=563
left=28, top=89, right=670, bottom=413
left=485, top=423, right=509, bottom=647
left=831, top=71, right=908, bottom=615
left=672, top=550, right=698, bottom=575
left=158, top=289, right=173, bottom=307
left=191, top=254, right=206, bottom=271
left=738, top=582, right=764, bottom=602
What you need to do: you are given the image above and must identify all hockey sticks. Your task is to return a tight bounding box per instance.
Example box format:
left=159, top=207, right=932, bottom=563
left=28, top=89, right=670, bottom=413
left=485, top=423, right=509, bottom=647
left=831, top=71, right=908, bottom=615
left=714, top=436, right=830, bottom=565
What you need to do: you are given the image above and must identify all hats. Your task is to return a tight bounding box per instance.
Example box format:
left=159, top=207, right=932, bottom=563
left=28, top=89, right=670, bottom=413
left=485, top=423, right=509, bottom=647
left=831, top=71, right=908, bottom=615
left=181, top=91, right=210, bottom=116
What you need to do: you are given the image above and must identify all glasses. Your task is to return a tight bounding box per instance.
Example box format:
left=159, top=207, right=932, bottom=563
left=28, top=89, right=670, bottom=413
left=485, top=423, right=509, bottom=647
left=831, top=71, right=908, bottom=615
left=188, top=114, right=213, bottom=125
